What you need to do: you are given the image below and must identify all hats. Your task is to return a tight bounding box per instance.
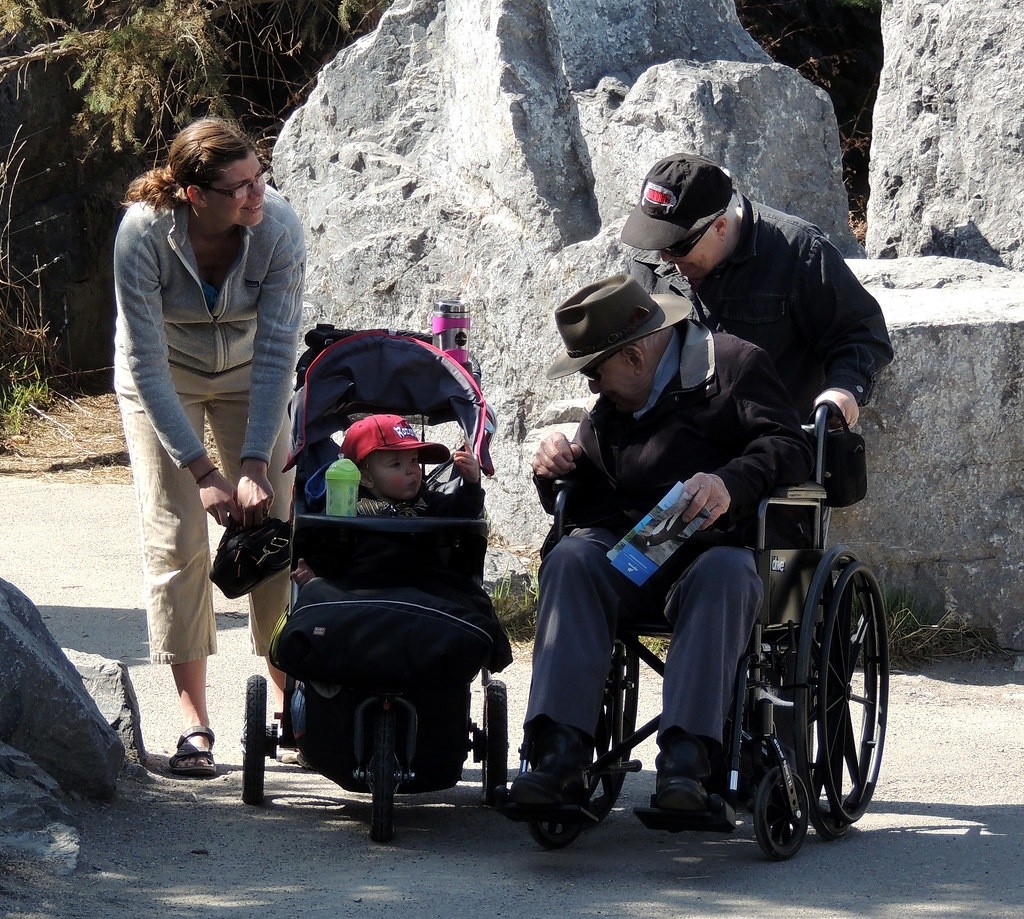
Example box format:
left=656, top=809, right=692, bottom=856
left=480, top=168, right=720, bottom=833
left=341, top=414, right=451, bottom=464
left=620, top=154, right=732, bottom=251
left=546, top=274, right=693, bottom=380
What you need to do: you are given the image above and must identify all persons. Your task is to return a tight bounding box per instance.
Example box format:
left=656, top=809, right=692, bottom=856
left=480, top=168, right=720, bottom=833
left=113, top=117, right=307, bottom=778
left=620, top=151, right=898, bottom=817
left=509, top=272, right=816, bottom=814
left=339, top=410, right=488, bottom=520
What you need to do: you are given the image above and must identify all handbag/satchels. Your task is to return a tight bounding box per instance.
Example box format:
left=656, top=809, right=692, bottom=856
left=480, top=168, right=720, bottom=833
left=209, top=510, right=293, bottom=599
left=795, top=402, right=868, bottom=509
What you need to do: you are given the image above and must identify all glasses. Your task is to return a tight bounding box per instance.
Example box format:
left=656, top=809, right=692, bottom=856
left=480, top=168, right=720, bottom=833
left=579, top=344, right=638, bottom=382
left=209, top=164, right=273, bottom=199
left=661, top=209, right=726, bottom=258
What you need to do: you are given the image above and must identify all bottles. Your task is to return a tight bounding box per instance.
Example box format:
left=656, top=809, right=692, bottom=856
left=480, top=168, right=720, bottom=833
left=326, top=459, right=360, bottom=517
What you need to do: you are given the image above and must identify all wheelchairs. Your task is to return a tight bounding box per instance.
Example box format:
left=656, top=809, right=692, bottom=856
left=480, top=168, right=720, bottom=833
left=508, top=400, right=890, bottom=860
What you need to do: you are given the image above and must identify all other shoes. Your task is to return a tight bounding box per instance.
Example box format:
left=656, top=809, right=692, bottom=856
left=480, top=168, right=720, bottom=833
left=511, top=753, right=589, bottom=803
left=655, top=736, right=710, bottom=809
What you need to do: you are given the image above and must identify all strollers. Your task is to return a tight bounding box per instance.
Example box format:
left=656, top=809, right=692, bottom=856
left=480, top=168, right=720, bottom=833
left=241, top=321, right=509, bottom=846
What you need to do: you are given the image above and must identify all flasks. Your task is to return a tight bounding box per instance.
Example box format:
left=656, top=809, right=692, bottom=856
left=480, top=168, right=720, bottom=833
left=433, top=300, right=470, bottom=364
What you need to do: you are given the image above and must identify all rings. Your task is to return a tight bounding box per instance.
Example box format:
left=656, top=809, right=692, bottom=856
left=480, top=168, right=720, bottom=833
left=263, top=509, right=271, bottom=516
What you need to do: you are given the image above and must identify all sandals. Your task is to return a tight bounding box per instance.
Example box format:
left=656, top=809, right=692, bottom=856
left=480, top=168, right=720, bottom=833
left=168, top=726, right=217, bottom=777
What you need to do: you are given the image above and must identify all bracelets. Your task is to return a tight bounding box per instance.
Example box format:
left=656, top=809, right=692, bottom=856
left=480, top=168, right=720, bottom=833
left=195, top=467, right=219, bottom=485
